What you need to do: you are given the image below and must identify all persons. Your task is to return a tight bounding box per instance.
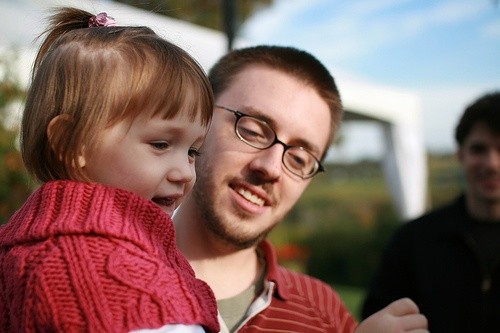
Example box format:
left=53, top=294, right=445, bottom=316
left=0, top=8, right=431, bottom=333
left=172, top=46, right=359, bottom=333
left=359, top=92, right=500, bottom=333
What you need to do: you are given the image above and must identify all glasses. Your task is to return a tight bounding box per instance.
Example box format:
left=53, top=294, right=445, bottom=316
left=214, top=105, right=326, bottom=180
